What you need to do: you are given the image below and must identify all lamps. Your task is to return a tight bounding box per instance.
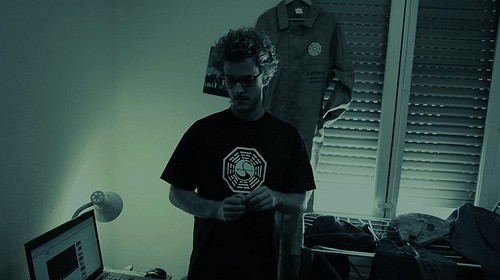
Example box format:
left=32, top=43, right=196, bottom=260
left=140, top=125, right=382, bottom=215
left=72, top=190, right=123, bottom=223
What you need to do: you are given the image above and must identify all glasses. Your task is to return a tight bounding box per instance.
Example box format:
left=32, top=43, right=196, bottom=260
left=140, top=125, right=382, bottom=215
left=218, top=73, right=262, bottom=90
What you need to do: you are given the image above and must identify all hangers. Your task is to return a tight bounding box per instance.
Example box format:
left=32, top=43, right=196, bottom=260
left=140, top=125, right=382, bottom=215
left=285, top=0, right=312, bottom=20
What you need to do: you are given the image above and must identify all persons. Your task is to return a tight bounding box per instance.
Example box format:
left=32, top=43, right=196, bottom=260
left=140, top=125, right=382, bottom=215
left=160, top=27, right=317, bottom=280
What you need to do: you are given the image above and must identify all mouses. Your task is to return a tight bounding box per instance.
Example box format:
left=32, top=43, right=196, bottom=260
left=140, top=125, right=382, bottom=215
left=146, top=267, right=166, bottom=279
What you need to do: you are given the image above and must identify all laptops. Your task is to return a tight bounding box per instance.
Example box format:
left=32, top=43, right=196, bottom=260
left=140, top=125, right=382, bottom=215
left=22, top=209, right=159, bottom=280
